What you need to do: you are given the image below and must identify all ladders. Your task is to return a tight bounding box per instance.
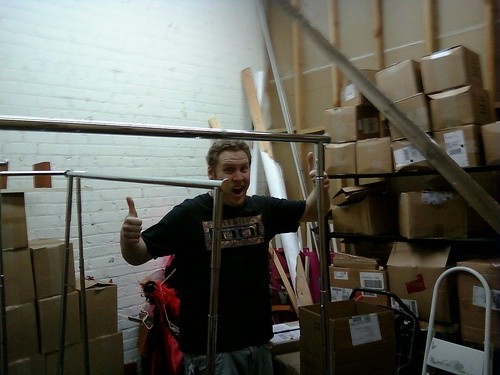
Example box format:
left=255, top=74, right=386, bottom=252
left=421, top=266, right=492, bottom=375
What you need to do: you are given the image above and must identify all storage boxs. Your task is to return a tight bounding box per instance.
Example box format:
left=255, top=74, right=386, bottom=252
left=272, top=44, right=500, bottom=375
left=0, top=189, right=124, bottom=375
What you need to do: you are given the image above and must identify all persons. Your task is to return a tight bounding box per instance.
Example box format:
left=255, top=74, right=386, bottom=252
left=120, top=139, right=331, bottom=375
